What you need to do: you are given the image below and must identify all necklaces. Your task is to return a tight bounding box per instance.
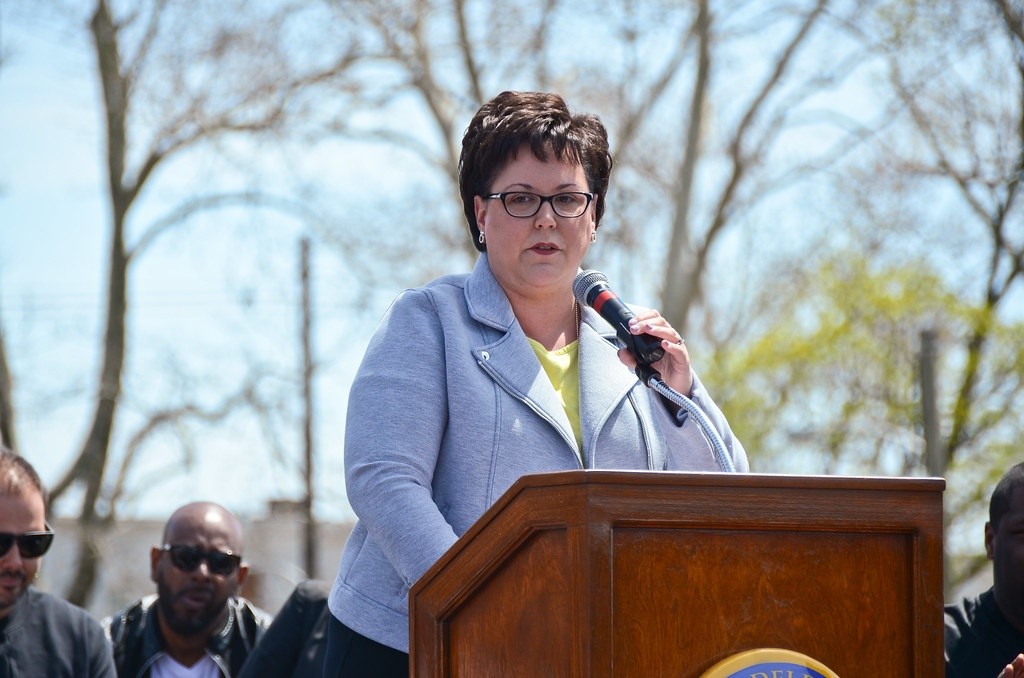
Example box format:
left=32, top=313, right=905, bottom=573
left=575, top=299, right=579, bottom=340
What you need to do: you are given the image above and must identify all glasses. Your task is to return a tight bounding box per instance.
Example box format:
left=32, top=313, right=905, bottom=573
left=159, top=540, right=242, bottom=577
left=481, top=191, right=594, bottom=218
left=0, top=523, right=55, bottom=558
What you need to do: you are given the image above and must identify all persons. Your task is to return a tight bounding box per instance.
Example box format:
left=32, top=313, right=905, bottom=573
left=944, top=461, right=1024, bottom=678
left=324, top=91, right=751, bottom=677
left=0, top=446, right=117, bottom=678
left=101, top=501, right=276, bottom=678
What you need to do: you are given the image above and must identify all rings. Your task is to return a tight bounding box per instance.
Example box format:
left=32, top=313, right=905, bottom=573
left=676, top=338, right=684, bottom=345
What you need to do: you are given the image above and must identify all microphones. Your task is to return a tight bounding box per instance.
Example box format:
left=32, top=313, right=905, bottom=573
left=574, top=268, right=666, bottom=364
